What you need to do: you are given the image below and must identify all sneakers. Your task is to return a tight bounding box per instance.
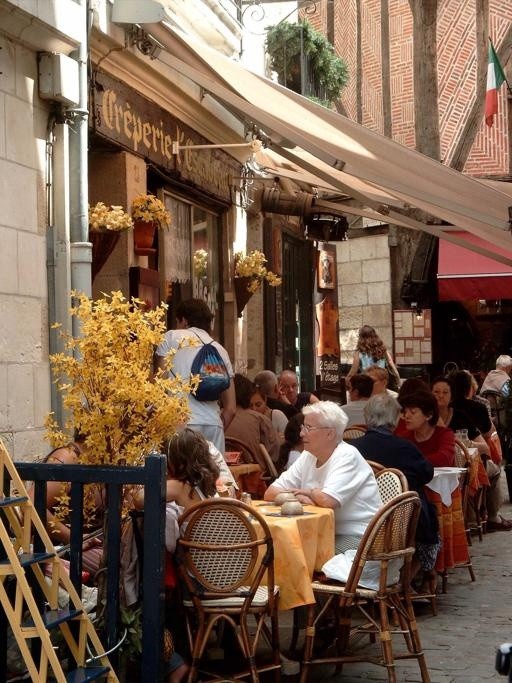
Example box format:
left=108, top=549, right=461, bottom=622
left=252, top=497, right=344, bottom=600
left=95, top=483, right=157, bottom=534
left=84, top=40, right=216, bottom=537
left=7, top=669, right=30, bottom=682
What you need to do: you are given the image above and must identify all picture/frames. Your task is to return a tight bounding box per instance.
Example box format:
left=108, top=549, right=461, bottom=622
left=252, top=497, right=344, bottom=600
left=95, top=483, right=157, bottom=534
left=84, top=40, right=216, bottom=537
left=316, top=248, right=339, bottom=292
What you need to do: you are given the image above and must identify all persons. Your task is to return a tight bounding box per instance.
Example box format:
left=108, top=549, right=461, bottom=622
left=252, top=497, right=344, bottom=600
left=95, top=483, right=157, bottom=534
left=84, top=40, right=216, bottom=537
left=13, top=299, right=512, bottom=683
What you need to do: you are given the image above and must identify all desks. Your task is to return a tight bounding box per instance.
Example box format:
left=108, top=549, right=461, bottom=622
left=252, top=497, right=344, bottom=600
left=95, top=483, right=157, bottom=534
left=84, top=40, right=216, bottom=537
left=235, top=500, right=338, bottom=651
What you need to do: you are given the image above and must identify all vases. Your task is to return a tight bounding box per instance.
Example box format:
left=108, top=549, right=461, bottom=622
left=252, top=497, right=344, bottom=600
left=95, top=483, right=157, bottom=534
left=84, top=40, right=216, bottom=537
left=87, top=228, right=122, bottom=287
left=234, top=273, right=262, bottom=318
left=133, top=217, right=159, bottom=249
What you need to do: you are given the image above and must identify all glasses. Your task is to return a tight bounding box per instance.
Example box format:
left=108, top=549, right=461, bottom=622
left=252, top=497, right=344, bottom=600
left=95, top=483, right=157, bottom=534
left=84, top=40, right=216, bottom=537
left=301, top=423, right=333, bottom=432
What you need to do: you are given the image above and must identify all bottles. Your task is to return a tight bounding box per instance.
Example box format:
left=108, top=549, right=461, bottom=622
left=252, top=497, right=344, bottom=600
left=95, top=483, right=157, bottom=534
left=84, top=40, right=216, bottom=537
left=247, top=494, right=252, bottom=508
left=241, top=492, right=248, bottom=503
left=455, top=427, right=469, bottom=450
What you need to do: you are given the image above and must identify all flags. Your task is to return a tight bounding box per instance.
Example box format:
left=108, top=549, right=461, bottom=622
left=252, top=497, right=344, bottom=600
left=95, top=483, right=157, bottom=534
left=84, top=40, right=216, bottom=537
left=485, top=41, right=506, bottom=128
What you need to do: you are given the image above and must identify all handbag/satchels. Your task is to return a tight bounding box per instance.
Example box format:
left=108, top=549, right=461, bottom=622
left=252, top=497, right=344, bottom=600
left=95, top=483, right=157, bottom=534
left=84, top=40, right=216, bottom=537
left=386, top=365, right=400, bottom=392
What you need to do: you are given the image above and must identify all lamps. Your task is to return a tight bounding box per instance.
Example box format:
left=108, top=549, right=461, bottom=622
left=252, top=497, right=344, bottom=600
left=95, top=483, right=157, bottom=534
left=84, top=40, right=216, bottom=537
left=170, top=139, right=271, bottom=167
left=301, top=210, right=350, bottom=241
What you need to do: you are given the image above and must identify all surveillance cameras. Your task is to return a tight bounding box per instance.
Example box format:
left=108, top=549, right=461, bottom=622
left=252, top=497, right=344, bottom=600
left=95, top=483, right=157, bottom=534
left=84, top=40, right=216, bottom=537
left=411, top=302, right=421, bottom=316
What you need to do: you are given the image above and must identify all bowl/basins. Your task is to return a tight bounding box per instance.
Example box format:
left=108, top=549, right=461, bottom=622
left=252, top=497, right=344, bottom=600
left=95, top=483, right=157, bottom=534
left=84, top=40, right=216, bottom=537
left=274, top=490, right=297, bottom=505
left=281, top=498, right=305, bottom=515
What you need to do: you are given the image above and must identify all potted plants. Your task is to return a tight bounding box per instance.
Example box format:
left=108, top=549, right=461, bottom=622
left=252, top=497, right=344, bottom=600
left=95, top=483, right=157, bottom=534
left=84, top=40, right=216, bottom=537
left=266, top=20, right=351, bottom=102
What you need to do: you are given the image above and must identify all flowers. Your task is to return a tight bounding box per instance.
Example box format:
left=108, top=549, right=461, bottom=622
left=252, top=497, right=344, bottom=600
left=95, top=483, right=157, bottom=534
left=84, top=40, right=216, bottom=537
left=88, top=200, right=133, bottom=232
left=235, top=249, right=284, bottom=293
left=133, top=190, right=173, bottom=229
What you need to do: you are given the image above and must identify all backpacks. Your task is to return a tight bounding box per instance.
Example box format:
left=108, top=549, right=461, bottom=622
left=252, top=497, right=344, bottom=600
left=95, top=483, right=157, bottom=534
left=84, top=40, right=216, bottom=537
left=169, top=331, right=230, bottom=401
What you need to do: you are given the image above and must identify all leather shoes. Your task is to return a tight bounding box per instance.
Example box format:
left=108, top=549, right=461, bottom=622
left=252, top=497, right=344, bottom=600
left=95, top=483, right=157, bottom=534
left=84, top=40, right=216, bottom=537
left=486, top=515, right=512, bottom=532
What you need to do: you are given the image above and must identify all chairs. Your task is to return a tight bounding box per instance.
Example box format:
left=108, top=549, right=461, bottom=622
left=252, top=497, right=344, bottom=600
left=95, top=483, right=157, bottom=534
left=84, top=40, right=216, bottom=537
left=363, top=468, right=410, bottom=647
left=226, top=386, right=512, bottom=593
left=295, top=490, right=432, bottom=682
left=170, top=494, right=283, bottom=682
